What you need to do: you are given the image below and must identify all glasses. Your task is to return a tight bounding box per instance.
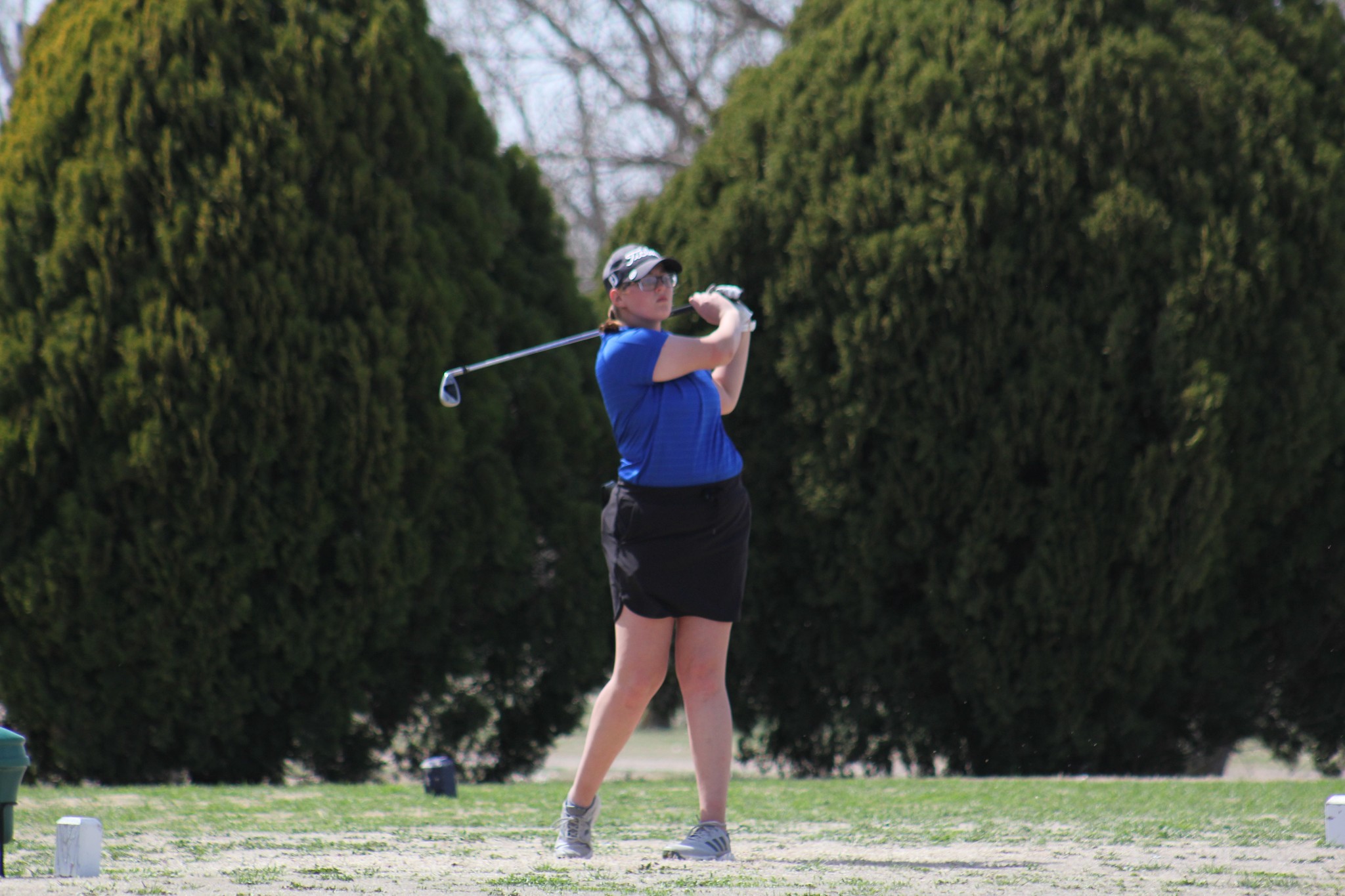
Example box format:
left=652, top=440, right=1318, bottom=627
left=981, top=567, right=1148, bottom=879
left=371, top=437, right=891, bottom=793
left=618, top=271, right=677, bottom=292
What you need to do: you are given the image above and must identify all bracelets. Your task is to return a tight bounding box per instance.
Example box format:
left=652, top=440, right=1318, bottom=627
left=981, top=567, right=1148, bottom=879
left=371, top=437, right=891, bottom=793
left=739, top=321, right=758, bottom=333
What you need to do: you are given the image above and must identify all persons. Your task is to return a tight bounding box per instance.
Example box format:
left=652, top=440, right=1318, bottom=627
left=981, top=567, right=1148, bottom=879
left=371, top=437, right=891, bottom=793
left=556, top=241, right=758, bottom=863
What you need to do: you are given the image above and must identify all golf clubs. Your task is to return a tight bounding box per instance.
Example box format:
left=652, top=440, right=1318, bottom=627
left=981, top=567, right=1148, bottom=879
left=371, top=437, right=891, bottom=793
left=439, top=305, right=695, bottom=409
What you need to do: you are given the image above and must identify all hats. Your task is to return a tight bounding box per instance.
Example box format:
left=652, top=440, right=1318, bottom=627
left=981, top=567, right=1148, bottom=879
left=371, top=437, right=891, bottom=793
left=602, top=243, right=683, bottom=293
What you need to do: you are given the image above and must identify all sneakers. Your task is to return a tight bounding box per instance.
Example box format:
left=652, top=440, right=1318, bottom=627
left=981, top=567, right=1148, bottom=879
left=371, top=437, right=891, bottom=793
left=553, top=793, right=600, bottom=859
left=662, top=814, right=736, bottom=861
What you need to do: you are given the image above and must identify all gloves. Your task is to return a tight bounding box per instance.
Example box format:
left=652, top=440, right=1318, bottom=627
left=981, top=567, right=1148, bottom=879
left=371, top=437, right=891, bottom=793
left=705, top=284, right=757, bottom=333
